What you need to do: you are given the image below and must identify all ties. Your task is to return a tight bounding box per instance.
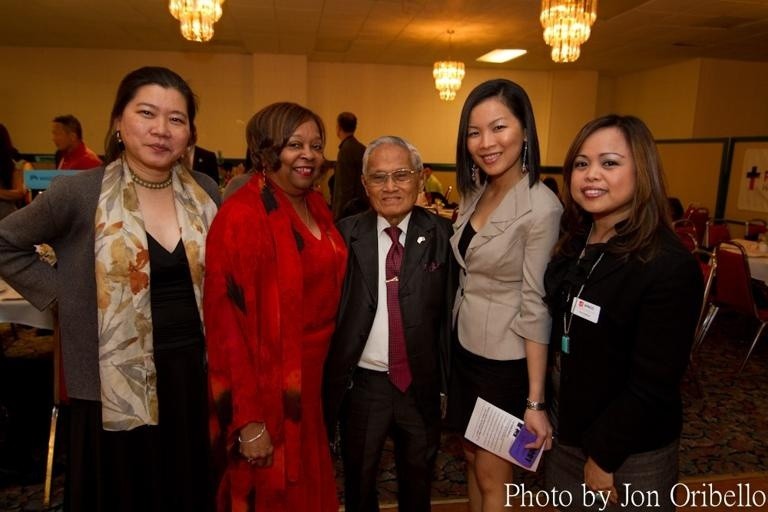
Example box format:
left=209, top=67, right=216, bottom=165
left=383, top=227, right=414, bottom=393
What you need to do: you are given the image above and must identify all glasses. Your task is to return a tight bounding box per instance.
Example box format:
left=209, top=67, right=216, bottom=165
left=366, top=168, right=419, bottom=185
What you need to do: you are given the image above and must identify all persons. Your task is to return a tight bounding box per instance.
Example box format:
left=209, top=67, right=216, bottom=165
left=0, top=64, right=704, bottom=512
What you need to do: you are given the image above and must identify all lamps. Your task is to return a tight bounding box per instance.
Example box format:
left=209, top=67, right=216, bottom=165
left=167, top=0, right=225, bottom=43
left=432, top=30, right=466, bottom=102
left=539, top=0, right=598, bottom=64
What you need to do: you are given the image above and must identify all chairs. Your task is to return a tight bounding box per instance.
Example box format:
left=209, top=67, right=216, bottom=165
left=421, top=190, right=459, bottom=222
left=542, top=175, right=565, bottom=208
left=672, top=202, right=768, bottom=386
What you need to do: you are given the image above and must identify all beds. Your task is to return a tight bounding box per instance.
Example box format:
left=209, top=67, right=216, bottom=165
left=0, top=243, right=58, bottom=339
